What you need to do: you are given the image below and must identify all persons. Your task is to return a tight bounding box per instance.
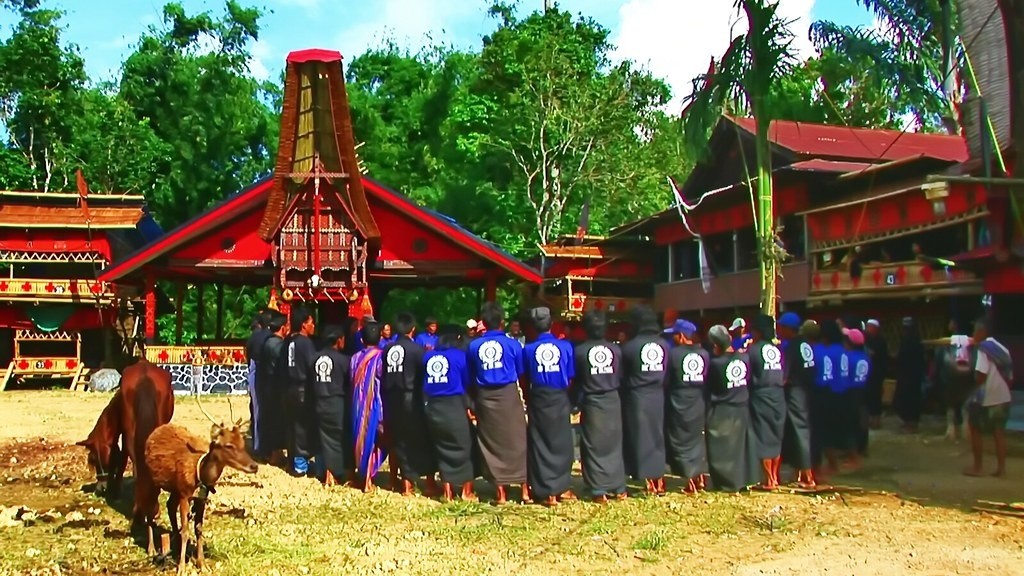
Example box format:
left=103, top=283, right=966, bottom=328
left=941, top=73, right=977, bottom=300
left=243, top=306, right=885, bottom=506
left=958, top=320, right=1014, bottom=477
left=892, top=305, right=987, bottom=437
left=822, top=239, right=924, bottom=269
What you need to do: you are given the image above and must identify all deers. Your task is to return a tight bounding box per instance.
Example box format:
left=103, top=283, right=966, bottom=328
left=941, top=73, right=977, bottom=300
left=143, top=388, right=258, bottom=576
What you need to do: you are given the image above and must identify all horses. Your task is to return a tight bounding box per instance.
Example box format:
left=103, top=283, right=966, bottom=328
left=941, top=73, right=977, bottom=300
left=75, top=360, right=175, bottom=531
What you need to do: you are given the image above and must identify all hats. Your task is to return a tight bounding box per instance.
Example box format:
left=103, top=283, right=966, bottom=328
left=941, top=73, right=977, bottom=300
left=359, top=314, right=377, bottom=326
left=466, top=318, right=478, bottom=329
left=867, top=318, right=880, bottom=327
left=901, top=317, right=913, bottom=327
left=797, top=320, right=822, bottom=337
left=842, top=327, right=865, bottom=345
left=663, top=318, right=697, bottom=339
left=728, top=317, right=746, bottom=331
left=774, top=312, right=802, bottom=330
